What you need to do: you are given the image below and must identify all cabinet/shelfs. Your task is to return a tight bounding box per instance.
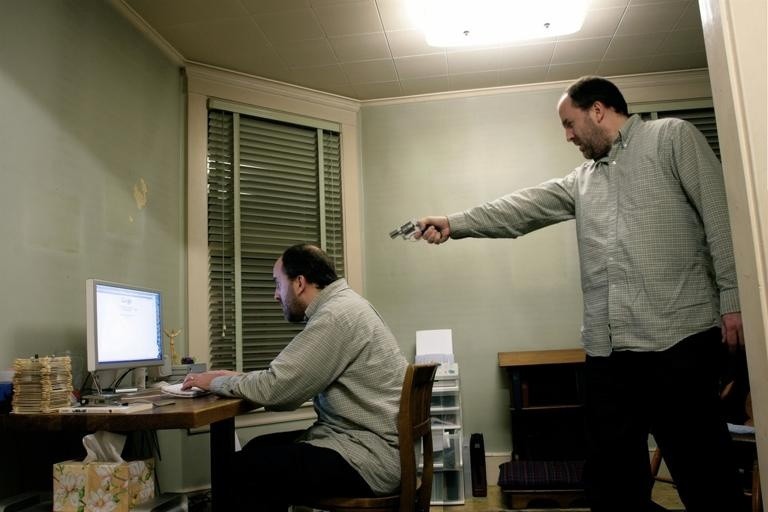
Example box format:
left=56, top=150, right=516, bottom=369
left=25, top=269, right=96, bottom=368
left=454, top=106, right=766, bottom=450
left=419, top=365, right=465, bottom=505
left=496, top=348, right=588, bottom=510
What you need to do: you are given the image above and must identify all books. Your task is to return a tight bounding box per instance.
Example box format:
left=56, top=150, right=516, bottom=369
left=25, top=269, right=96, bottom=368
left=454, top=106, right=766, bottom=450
left=59, top=403, right=154, bottom=415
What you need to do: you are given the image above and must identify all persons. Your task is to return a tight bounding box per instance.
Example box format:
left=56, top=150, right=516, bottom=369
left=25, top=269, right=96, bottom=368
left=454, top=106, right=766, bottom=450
left=416, top=76, right=744, bottom=511
left=181, top=244, right=406, bottom=512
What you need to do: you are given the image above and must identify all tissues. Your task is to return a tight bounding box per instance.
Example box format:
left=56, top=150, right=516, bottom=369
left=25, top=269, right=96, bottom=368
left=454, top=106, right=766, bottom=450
left=53, top=430, right=156, bottom=512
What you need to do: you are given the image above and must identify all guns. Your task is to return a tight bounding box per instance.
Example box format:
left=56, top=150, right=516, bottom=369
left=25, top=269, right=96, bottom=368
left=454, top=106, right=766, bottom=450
left=389, top=219, right=422, bottom=240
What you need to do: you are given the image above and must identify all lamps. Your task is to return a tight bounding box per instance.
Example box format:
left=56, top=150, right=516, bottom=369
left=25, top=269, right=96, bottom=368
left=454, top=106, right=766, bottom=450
left=401, top=2, right=591, bottom=47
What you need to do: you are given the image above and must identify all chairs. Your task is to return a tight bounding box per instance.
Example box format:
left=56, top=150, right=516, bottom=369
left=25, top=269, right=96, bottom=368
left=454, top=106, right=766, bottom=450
left=288, top=363, right=439, bottom=511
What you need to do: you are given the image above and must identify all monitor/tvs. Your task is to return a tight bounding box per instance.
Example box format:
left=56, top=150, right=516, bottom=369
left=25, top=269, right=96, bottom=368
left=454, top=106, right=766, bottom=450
left=82, top=278, right=165, bottom=398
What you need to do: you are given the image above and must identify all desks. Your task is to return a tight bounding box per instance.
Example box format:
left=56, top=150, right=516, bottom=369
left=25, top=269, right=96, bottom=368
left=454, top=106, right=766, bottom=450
left=10, top=392, right=260, bottom=510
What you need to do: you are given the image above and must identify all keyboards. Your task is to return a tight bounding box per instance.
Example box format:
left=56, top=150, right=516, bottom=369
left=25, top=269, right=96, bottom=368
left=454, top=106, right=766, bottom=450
left=161, top=383, right=211, bottom=398
